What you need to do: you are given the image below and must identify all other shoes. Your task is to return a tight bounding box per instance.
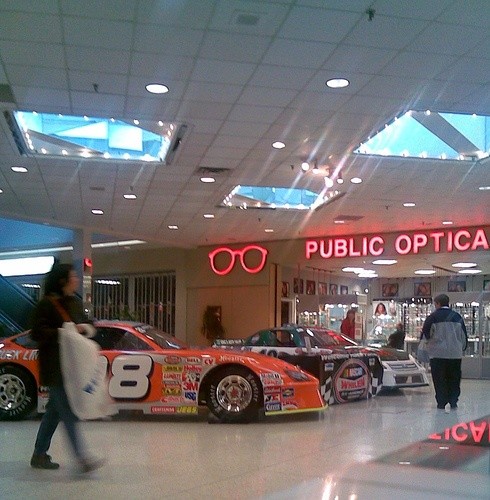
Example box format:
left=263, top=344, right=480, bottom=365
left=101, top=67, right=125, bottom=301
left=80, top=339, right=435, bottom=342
left=450, top=402, right=457, bottom=408
left=438, top=403, right=445, bottom=409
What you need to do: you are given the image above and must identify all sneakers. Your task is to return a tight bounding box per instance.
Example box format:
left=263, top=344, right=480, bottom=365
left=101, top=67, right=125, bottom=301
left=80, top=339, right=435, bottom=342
left=30, top=454, right=60, bottom=470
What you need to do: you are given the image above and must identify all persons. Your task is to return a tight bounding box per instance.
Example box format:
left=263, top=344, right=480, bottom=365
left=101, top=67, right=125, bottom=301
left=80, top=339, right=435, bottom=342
left=375, top=303, right=387, bottom=316
left=30, top=263, right=105, bottom=474
left=422, top=293, right=468, bottom=409
left=283, top=279, right=464, bottom=297
left=340, top=310, right=355, bottom=340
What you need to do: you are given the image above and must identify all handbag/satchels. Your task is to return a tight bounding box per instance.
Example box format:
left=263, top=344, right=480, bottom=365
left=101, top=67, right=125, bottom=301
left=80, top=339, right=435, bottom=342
left=417, top=333, right=429, bottom=363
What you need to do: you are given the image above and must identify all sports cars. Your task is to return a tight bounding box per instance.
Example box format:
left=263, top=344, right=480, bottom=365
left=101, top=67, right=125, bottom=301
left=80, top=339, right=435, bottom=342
left=0, top=318, right=329, bottom=423
left=213, top=323, right=431, bottom=390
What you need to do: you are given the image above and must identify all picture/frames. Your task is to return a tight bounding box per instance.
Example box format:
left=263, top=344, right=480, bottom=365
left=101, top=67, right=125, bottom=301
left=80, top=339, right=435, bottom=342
left=329, top=284, right=339, bottom=295
left=317, top=282, right=328, bottom=295
left=294, top=278, right=304, bottom=294
left=448, top=281, right=466, bottom=292
left=482, top=280, right=490, bottom=291
left=306, top=280, right=317, bottom=295
left=382, top=283, right=399, bottom=297
left=414, top=282, right=432, bottom=296
left=281, top=282, right=291, bottom=297
left=340, top=285, right=348, bottom=295
